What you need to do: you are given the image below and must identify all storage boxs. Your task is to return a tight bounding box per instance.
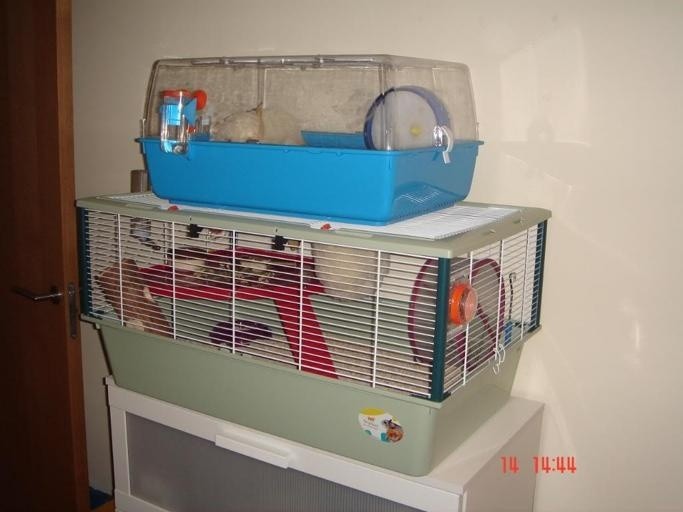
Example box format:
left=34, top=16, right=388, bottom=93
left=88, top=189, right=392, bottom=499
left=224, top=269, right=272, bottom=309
left=79, top=313, right=543, bottom=478
left=135, top=53, right=484, bottom=227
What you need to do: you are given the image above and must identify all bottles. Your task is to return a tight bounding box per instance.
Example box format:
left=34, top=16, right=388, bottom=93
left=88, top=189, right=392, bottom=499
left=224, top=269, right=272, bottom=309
left=159, top=87, right=193, bottom=155
